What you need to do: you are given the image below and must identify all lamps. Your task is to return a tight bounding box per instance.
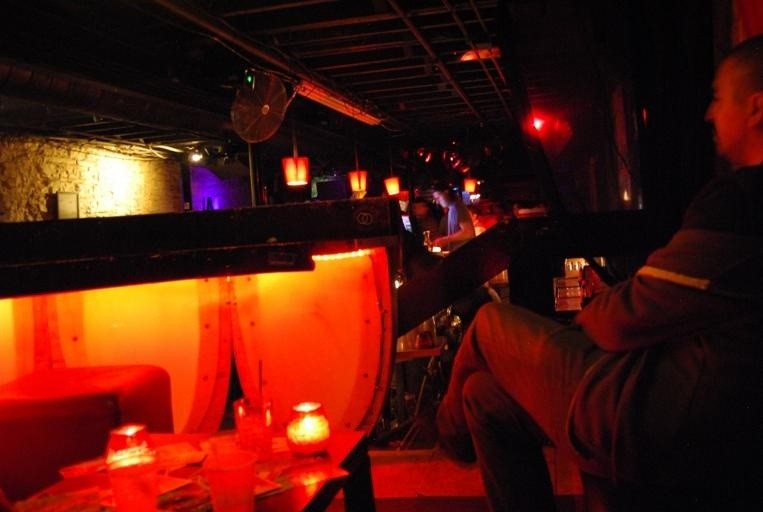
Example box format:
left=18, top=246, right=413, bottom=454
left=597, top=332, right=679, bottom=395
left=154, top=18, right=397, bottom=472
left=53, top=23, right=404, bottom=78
left=346, top=141, right=370, bottom=195
left=278, top=121, right=313, bottom=189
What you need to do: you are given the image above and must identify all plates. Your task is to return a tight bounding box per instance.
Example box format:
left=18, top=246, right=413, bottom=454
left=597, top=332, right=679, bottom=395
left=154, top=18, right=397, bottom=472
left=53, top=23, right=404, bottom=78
left=101, top=475, right=193, bottom=508
left=198, top=474, right=282, bottom=497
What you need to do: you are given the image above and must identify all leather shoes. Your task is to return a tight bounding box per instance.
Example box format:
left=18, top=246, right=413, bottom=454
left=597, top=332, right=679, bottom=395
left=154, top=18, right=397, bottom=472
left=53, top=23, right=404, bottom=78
left=439, top=424, right=477, bottom=465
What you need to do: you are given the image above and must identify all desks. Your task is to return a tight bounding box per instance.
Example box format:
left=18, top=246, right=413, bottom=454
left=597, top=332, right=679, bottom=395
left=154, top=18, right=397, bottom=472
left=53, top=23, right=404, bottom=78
left=14, top=427, right=368, bottom=512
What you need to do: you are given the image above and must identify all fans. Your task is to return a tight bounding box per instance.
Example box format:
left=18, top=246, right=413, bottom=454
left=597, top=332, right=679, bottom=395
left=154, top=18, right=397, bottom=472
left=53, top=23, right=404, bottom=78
left=231, top=72, right=289, bottom=145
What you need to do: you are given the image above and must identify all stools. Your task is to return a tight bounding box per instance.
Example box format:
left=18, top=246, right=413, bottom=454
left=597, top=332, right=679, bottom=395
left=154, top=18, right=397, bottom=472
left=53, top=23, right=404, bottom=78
left=0, top=362, right=175, bottom=489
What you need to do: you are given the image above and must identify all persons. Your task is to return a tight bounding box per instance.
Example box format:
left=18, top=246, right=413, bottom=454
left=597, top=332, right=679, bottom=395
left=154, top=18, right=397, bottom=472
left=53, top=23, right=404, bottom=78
left=434, top=34, right=763, bottom=512
left=431, top=182, right=476, bottom=253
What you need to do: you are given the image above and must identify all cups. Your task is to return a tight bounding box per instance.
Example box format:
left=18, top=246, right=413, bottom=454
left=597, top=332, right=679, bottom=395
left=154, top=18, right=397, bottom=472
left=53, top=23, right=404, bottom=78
left=234, top=395, right=274, bottom=453
